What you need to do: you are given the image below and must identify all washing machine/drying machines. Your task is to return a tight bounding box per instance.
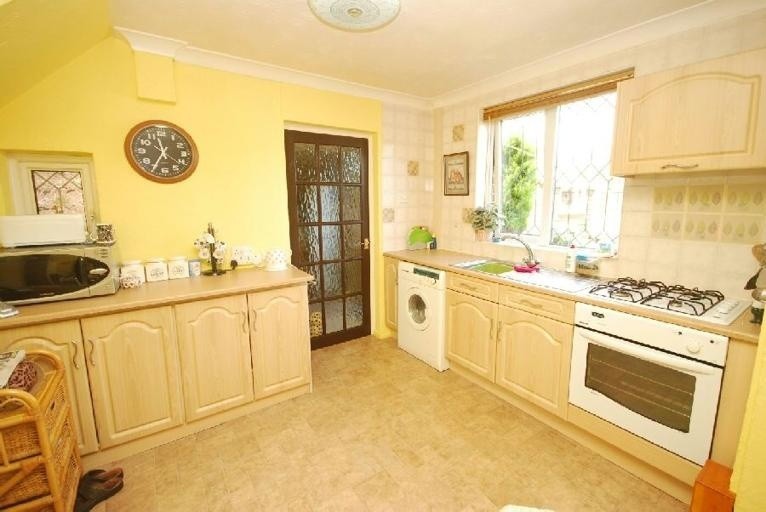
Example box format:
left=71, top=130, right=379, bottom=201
left=397, top=261, right=445, bottom=372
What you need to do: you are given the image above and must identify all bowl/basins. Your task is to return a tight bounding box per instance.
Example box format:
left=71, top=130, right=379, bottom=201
left=750, top=286, right=766, bottom=309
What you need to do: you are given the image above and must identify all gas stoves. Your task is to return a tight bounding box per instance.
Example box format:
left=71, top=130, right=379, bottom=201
left=573, top=274, right=754, bottom=326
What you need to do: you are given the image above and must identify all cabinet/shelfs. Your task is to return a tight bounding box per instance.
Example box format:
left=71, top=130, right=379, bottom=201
left=446, top=272, right=574, bottom=422
left=175, top=282, right=312, bottom=435
left=0, top=306, right=184, bottom=456
left=611, top=48, right=766, bottom=176
left=0, top=349, right=82, bottom=512
left=383, top=256, right=399, bottom=340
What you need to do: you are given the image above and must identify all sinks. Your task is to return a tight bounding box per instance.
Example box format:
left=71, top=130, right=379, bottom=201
left=453, top=259, right=515, bottom=276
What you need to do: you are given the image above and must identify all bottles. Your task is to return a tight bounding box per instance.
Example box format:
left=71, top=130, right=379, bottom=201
left=432, top=234, right=436, bottom=249
left=564, top=245, right=575, bottom=275
left=121, top=256, right=200, bottom=285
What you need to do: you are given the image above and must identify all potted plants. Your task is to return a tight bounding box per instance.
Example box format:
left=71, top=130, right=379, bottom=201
left=463, top=208, right=506, bottom=241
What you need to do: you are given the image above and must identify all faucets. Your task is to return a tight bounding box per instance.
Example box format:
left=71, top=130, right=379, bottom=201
left=501, top=235, right=542, bottom=268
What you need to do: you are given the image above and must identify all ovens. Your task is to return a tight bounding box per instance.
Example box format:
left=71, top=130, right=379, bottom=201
left=568, top=300, right=730, bottom=468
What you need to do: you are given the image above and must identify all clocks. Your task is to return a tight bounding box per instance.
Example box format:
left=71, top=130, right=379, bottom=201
left=123, top=120, right=199, bottom=184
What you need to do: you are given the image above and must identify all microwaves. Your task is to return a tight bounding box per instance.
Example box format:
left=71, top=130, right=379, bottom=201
left=1, top=241, right=119, bottom=307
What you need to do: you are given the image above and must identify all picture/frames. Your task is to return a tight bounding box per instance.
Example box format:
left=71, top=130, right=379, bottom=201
left=444, top=151, right=469, bottom=196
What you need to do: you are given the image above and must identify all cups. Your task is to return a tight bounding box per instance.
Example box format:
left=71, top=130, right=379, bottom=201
left=91, top=222, right=115, bottom=245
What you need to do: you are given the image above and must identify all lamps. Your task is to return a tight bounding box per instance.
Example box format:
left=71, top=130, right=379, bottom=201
left=307, top=0, right=401, bottom=34
